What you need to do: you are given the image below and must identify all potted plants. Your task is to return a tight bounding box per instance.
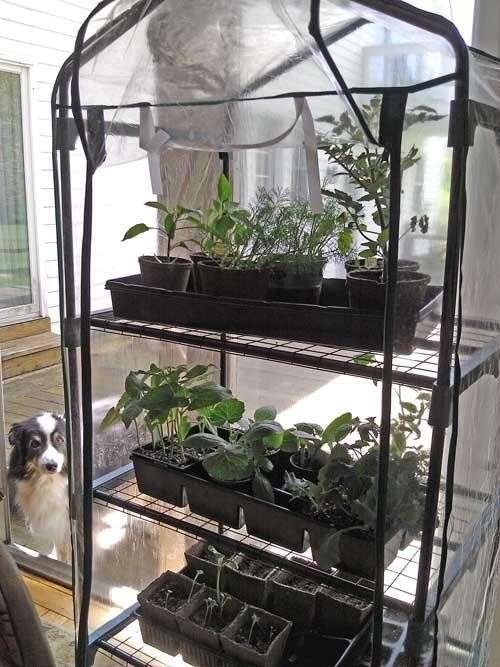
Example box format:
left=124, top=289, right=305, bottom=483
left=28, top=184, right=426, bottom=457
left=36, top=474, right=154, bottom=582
left=97, top=353, right=441, bottom=578
left=121, top=94, right=447, bottom=354
left=135, top=539, right=377, bottom=667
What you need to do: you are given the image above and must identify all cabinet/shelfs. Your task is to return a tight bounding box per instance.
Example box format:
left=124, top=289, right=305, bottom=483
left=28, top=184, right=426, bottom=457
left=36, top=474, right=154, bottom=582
left=48, top=1, right=500, bottom=667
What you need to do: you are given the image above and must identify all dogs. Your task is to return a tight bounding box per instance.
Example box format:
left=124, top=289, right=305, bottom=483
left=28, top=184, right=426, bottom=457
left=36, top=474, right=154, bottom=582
left=4, top=393, right=155, bottom=565
